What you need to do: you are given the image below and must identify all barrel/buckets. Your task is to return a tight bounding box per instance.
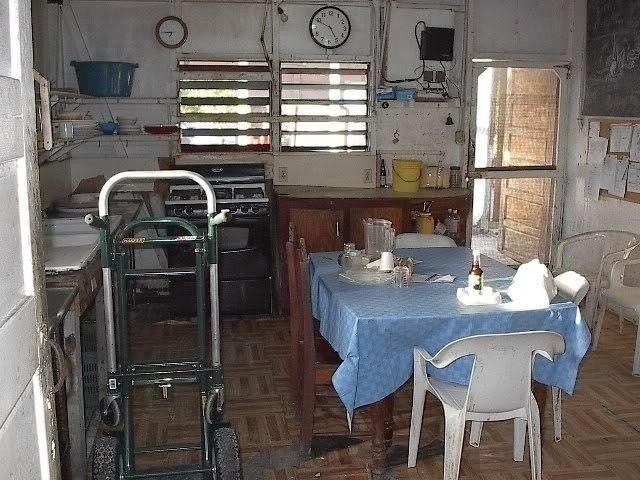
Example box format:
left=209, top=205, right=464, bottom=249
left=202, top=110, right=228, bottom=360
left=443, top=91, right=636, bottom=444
left=391, top=159, right=423, bottom=193
left=364, top=218, right=392, bottom=257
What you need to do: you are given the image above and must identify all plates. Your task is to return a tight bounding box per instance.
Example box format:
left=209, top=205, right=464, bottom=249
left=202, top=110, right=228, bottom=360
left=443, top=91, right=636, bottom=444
left=143, top=126, right=180, bottom=134
left=51, top=112, right=99, bottom=139
left=117, top=126, right=141, bottom=135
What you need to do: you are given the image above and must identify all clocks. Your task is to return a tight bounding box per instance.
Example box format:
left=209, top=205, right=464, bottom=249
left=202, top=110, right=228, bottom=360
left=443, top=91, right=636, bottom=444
left=308, top=5, right=353, bottom=50
left=155, top=15, right=189, bottom=49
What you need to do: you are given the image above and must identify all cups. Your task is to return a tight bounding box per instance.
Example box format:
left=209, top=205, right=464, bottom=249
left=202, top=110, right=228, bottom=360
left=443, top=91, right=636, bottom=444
left=59, top=122, right=74, bottom=139
left=393, top=266, right=411, bottom=289
left=343, top=242, right=362, bottom=276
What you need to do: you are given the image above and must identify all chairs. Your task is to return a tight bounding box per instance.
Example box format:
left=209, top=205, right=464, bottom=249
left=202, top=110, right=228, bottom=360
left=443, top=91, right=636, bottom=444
left=407, top=330, right=565, bottom=480
left=469, top=270, right=590, bottom=449
left=284, top=238, right=394, bottom=460
left=552, top=229, right=640, bottom=377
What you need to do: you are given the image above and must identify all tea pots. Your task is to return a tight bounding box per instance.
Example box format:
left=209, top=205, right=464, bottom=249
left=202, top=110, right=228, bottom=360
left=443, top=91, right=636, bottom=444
left=361, top=217, right=396, bottom=263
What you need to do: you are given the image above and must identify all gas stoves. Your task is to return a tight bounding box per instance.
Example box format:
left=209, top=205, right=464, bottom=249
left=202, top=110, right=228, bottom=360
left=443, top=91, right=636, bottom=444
left=165, top=186, right=269, bottom=202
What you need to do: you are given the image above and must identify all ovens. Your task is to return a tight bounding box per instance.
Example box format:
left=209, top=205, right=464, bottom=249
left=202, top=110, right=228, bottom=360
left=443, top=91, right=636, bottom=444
left=166, top=207, right=274, bottom=315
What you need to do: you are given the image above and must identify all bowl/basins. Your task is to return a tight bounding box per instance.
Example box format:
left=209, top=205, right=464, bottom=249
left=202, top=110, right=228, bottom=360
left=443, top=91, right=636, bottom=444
left=99, top=123, right=117, bottom=134
left=115, top=116, right=138, bottom=126
left=69, top=60, right=139, bottom=98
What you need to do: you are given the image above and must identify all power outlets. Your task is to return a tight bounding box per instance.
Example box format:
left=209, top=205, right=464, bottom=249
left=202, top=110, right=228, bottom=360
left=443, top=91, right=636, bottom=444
left=278, top=166, right=288, bottom=183
left=362, top=168, right=372, bottom=184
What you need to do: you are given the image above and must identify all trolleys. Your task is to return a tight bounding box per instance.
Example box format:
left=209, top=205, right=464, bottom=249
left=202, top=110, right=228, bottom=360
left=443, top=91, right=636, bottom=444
left=85, top=168, right=242, bottom=479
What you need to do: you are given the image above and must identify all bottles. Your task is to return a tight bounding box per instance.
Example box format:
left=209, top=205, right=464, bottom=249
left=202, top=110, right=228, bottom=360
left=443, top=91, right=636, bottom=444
left=444, top=208, right=460, bottom=241
left=450, top=166, right=461, bottom=188
left=467, top=249, right=483, bottom=296
left=380, top=159, right=386, bottom=188
left=414, top=212, right=435, bottom=234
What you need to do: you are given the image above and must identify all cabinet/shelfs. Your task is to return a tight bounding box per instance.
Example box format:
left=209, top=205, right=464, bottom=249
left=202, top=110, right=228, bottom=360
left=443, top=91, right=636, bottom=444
left=273, top=194, right=472, bottom=315
left=56, top=98, right=179, bottom=143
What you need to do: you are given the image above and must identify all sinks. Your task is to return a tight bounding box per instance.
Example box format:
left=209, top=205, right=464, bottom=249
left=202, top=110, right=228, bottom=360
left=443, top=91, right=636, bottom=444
left=44, top=233, right=100, bottom=271
left=42, top=216, right=122, bottom=234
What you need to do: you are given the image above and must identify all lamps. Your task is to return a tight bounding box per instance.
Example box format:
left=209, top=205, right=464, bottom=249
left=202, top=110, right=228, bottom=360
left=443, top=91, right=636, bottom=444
left=276, top=6, right=288, bottom=23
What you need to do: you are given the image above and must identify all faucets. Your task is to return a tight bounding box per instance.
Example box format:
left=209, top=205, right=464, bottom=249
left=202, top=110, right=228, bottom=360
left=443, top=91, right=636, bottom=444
left=40, top=210, right=48, bottom=220
